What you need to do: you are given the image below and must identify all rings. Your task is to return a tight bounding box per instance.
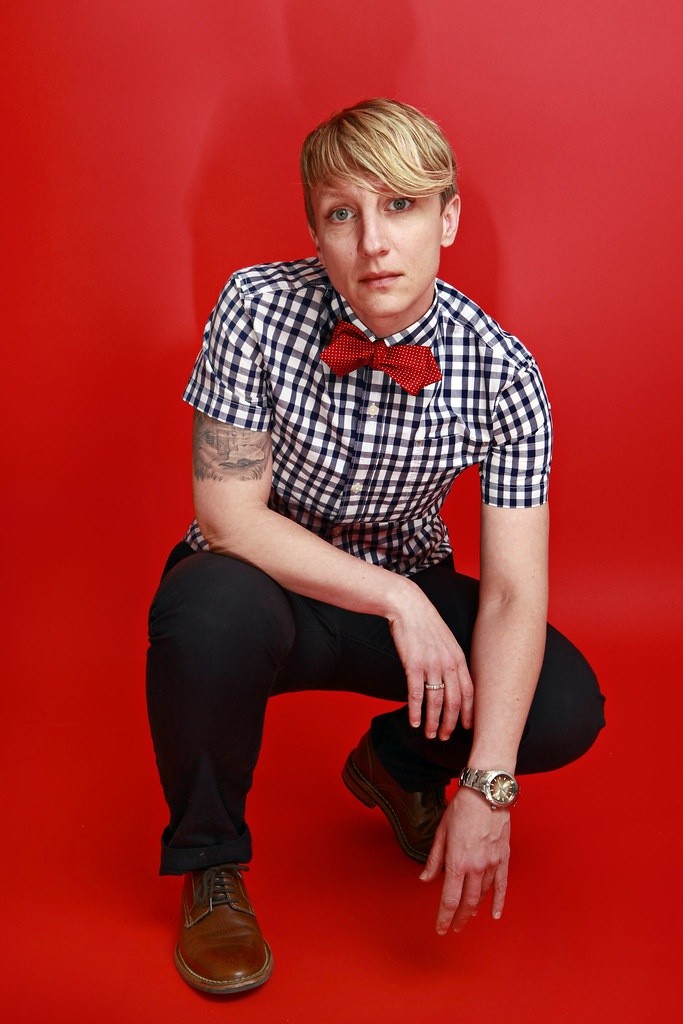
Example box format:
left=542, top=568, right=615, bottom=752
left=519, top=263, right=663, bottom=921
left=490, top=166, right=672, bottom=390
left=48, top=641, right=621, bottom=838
left=424, top=682, right=445, bottom=690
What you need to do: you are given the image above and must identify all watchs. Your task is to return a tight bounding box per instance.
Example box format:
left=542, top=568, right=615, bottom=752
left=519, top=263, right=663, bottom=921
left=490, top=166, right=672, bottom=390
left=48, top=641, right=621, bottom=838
left=457, top=766, right=522, bottom=810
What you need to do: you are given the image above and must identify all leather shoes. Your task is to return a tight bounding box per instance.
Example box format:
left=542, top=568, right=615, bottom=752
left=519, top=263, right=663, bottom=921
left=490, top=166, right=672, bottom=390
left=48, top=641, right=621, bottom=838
left=174, top=861, right=272, bottom=993
left=342, top=729, right=449, bottom=867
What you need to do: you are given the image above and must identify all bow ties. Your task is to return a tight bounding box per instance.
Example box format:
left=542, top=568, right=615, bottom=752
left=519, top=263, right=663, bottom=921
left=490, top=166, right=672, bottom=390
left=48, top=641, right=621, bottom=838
left=319, top=320, right=442, bottom=395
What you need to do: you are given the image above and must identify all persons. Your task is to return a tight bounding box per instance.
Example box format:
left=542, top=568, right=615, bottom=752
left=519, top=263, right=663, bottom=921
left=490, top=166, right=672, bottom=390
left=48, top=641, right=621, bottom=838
left=144, top=97, right=608, bottom=1002
